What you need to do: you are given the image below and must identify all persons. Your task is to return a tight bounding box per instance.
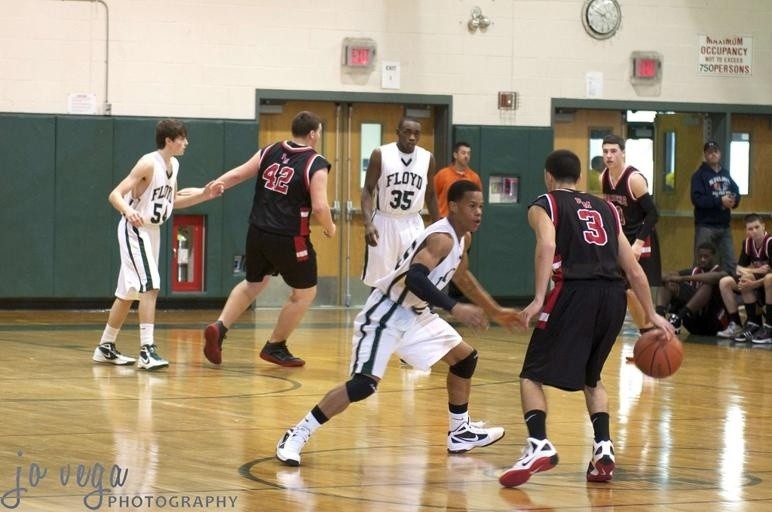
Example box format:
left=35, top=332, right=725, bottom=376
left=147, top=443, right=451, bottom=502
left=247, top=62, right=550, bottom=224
left=598, top=134, right=662, bottom=359
left=752, top=273, right=772, bottom=344
left=276, top=180, right=529, bottom=467
left=93, top=120, right=225, bottom=372
left=589, top=157, right=606, bottom=195
left=178, top=111, right=336, bottom=367
left=716, top=214, right=772, bottom=342
left=361, top=116, right=440, bottom=364
left=433, top=143, right=482, bottom=318
left=691, top=141, right=747, bottom=318
left=499, top=150, right=676, bottom=487
left=656, top=243, right=732, bottom=337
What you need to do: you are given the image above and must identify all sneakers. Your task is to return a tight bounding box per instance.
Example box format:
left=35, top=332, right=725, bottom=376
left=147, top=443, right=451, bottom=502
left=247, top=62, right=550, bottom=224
left=202, top=319, right=229, bottom=365
left=136, top=344, right=170, bottom=372
left=446, top=415, right=505, bottom=455
left=664, top=313, right=682, bottom=335
left=91, top=341, right=136, bottom=367
left=716, top=321, right=772, bottom=344
left=586, top=438, right=617, bottom=482
left=275, top=424, right=311, bottom=467
left=259, top=340, right=306, bottom=368
left=498, top=436, right=559, bottom=488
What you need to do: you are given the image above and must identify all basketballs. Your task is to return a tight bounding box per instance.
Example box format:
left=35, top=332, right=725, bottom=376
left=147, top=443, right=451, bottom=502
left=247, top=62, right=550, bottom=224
left=634, top=328, right=684, bottom=377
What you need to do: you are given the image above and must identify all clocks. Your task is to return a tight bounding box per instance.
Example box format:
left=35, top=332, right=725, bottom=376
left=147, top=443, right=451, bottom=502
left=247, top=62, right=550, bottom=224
left=581, top=0, right=622, bottom=40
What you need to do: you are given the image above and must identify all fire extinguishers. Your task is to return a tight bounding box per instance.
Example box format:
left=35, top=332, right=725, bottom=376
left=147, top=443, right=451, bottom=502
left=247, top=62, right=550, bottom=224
left=177, top=225, right=189, bottom=282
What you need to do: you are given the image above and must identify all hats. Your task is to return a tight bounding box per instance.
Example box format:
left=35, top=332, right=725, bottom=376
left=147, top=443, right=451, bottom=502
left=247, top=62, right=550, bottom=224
left=703, top=140, right=719, bottom=153
